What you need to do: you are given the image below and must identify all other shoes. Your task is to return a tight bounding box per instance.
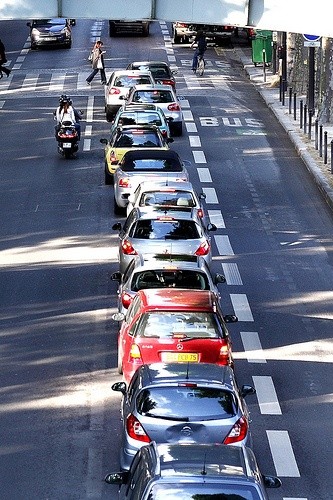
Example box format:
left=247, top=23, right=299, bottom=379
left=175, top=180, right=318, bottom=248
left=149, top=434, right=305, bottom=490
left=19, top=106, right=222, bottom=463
left=86, top=81, right=90, bottom=86
left=7, top=71, right=11, bottom=77
left=191, top=68, right=196, bottom=70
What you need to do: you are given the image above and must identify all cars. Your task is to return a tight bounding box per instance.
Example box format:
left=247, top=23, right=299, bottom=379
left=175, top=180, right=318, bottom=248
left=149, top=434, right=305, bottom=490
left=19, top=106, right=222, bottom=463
left=112, top=205, right=217, bottom=274
left=110, top=103, right=175, bottom=150
left=110, top=253, right=226, bottom=330
left=70, top=19, right=76, bottom=25
left=99, top=123, right=174, bottom=185
left=121, top=180, right=206, bottom=222
left=111, top=149, right=192, bottom=215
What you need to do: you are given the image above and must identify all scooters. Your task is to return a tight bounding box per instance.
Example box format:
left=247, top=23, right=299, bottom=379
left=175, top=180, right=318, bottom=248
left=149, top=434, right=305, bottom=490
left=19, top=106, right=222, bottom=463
left=53, top=109, right=84, bottom=159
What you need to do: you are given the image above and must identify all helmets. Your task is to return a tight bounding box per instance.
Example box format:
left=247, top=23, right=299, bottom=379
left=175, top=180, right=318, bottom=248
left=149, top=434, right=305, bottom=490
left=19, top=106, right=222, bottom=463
left=59, top=94, right=68, bottom=102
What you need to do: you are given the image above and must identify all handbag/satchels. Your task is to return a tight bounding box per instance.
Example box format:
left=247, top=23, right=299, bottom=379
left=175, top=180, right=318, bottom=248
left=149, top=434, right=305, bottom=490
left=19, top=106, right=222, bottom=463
left=54, top=125, right=60, bottom=132
left=97, top=59, right=104, bottom=69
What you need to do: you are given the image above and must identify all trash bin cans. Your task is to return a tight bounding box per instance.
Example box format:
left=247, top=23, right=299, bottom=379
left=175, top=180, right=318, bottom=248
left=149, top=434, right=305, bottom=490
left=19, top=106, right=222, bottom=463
left=251, top=35, right=273, bottom=67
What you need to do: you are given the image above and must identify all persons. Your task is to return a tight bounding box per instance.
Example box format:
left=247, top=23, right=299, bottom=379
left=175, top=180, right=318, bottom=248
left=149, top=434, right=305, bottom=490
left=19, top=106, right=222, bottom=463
left=54, top=94, right=82, bottom=142
left=86, top=41, right=107, bottom=86
left=191, top=30, right=207, bottom=70
left=0, top=40, right=11, bottom=78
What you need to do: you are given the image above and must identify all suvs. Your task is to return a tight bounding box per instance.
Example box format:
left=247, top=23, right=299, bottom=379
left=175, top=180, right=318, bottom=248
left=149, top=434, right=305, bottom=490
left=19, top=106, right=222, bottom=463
left=111, top=288, right=238, bottom=384
left=109, top=20, right=149, bottom=37
left=126, top=61, right=178, bottom=94
left=111, top=363, right=256, bottom=473
left=26, top=19, right=73, bottom=50
left=105, top=441, right=283, bottom=500
left=118, top=83, right=182, bottom=137
left=101, top=70, right=160, bottom=122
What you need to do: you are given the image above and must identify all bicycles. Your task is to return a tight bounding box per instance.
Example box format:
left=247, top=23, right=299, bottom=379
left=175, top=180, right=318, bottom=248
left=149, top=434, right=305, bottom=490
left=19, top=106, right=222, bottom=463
left=189, top=46, right=206, bottom=76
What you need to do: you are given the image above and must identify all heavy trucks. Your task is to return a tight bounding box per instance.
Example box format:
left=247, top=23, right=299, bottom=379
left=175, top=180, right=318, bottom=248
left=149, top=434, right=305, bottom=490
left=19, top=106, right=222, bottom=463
left=170, top=22, right=236, bottom=44
